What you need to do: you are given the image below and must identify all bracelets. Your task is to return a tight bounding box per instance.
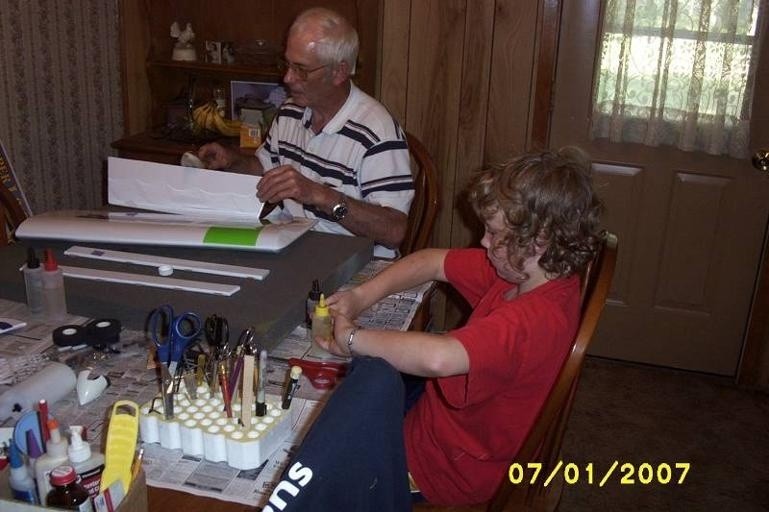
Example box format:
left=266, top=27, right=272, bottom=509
left=347, top=325, right=362, bottom=355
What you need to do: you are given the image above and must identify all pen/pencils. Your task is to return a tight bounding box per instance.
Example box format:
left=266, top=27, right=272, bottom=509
left=219, top=345, right=302, bottom=419
left=131, top=448, right=144, bottom=484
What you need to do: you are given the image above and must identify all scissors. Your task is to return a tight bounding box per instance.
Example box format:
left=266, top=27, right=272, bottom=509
left=265, top=356, right=346, bottom=389
left=152, top=304, right=257, bottom=419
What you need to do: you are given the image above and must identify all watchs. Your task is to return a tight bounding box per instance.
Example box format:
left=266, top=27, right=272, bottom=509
left=326, top=192, right=349, bottom=222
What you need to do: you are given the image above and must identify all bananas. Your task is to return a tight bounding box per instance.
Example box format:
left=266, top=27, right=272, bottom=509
left=193, top=100, right=244, bottom=136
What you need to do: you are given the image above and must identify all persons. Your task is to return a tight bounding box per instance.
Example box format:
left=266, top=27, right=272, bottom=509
left=259, top=145, right=603, bottom=512
left=193, top=6, right=416, bottom=263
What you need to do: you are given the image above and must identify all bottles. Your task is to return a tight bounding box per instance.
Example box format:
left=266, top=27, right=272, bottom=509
left=24, top=244, right=69, bottom=320
left=305, top=278, right=332, bottom=354
left=5, top=418, right=106, bottom=512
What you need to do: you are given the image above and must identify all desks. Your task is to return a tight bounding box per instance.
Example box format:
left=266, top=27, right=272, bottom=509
left=111, top=0, right=386, bottom=166
left=1, top=258, right=442, bottom=512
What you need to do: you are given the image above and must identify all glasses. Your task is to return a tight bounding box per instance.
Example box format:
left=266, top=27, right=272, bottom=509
left=276, top=54, right=344, bottom=82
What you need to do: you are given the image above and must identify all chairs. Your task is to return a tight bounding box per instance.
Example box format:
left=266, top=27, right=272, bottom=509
left=481, top=234, right=616, bottom=509
left=406, top=133, right=439, bottom=246
